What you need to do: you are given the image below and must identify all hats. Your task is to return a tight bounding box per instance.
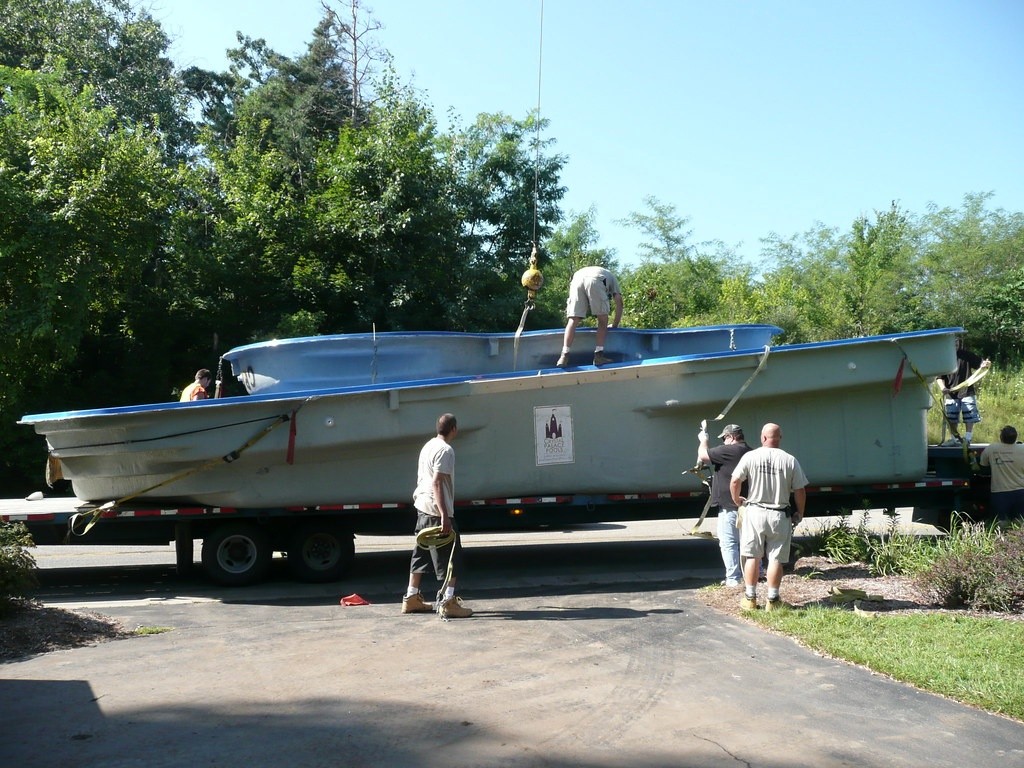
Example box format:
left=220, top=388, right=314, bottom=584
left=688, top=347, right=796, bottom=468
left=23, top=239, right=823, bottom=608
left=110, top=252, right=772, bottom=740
left=718, top=424, right=742, bottom=438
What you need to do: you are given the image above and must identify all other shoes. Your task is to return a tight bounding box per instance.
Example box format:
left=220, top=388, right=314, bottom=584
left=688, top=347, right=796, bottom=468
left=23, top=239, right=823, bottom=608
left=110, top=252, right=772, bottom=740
left=941, top=439, right=957, bottom=446
left=958, top=438, right=970, bottom=447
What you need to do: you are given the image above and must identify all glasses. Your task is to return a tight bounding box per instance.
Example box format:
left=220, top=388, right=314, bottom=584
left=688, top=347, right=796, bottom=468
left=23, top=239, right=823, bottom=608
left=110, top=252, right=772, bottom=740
left=722, top=435, right=729, bottom=441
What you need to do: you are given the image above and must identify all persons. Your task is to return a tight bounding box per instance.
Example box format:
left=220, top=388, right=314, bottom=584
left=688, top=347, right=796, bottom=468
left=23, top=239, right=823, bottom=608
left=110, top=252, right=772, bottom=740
left=730, top=422, right=810, bottom=611
left=980, top=425, right=1024, bottom=521
left=557, top=266, right=623, bottom=368
left=180, top=369, right=212, bottom=402
left=697, top=424, right=767, bottom=586
left=935, top=337, right=990, bottom=447
left=402, top=413, right=473, bottom=618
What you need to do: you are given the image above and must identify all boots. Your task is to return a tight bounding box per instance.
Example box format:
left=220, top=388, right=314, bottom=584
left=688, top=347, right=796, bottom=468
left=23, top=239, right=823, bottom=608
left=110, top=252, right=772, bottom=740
left=593, top=351, right=614, bottom=366
left=765, top=595, right=779, bottom=612
left=402, top=590, right=433, bottom=613
left=439, top=596, right=473, bottom=617
left=737, top=593, right=756, bottom=612
left=556, top=353, right=571, bottom=368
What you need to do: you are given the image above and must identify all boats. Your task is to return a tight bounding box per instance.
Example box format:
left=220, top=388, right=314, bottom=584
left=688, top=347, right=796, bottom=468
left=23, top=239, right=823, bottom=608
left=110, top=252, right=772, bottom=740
left=16, top=321, right=972, bottom=510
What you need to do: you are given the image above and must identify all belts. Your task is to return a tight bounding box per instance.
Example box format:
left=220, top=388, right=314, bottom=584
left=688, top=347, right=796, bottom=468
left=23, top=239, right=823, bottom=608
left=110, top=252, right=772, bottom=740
left=750, top=503, right=785, bottom=511
